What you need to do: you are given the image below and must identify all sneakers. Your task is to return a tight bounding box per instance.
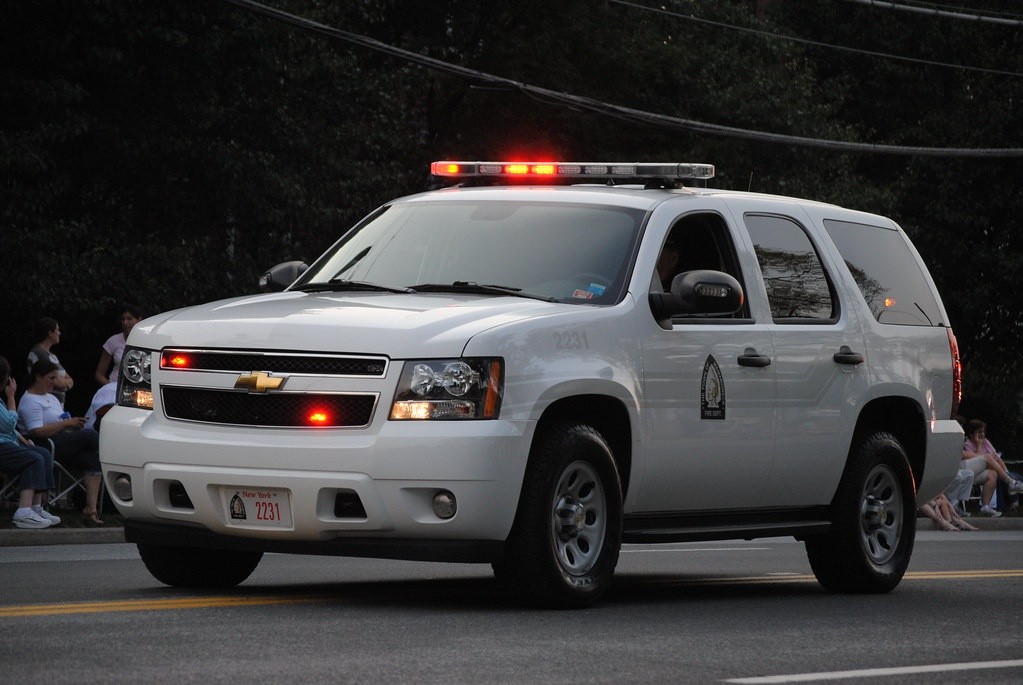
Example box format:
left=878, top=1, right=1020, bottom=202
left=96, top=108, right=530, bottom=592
left=982, top=508, right=1002, bottom=517
left=12, top=509, right=51, bottom=529
left=32, top=505, right=61, bottom=525
left=1009, top=480, right=1023, bottom=495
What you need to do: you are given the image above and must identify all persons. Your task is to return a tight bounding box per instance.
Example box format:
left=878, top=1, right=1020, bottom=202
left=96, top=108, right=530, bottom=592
left=1, top=304, right=146, bottom=531
left=917, top=415, right=1023, bottom=532
left=656, top=230, right=690, bottom=296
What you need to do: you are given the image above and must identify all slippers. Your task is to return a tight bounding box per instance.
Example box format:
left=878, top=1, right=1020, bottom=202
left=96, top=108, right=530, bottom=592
left=82, top=511, right=104, bottom=525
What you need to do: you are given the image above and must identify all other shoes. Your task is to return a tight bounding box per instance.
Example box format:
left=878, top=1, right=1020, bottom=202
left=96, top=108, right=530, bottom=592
left=955, top=507, right=971, bottom=516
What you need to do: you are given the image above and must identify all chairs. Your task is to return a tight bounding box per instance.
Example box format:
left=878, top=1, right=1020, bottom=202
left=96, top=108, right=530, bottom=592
left=0, top=435, right=104, bottom=521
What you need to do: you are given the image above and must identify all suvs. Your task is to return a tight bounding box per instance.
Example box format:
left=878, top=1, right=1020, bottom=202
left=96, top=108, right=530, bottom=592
left=94, top=158, right=965, bottom=612
